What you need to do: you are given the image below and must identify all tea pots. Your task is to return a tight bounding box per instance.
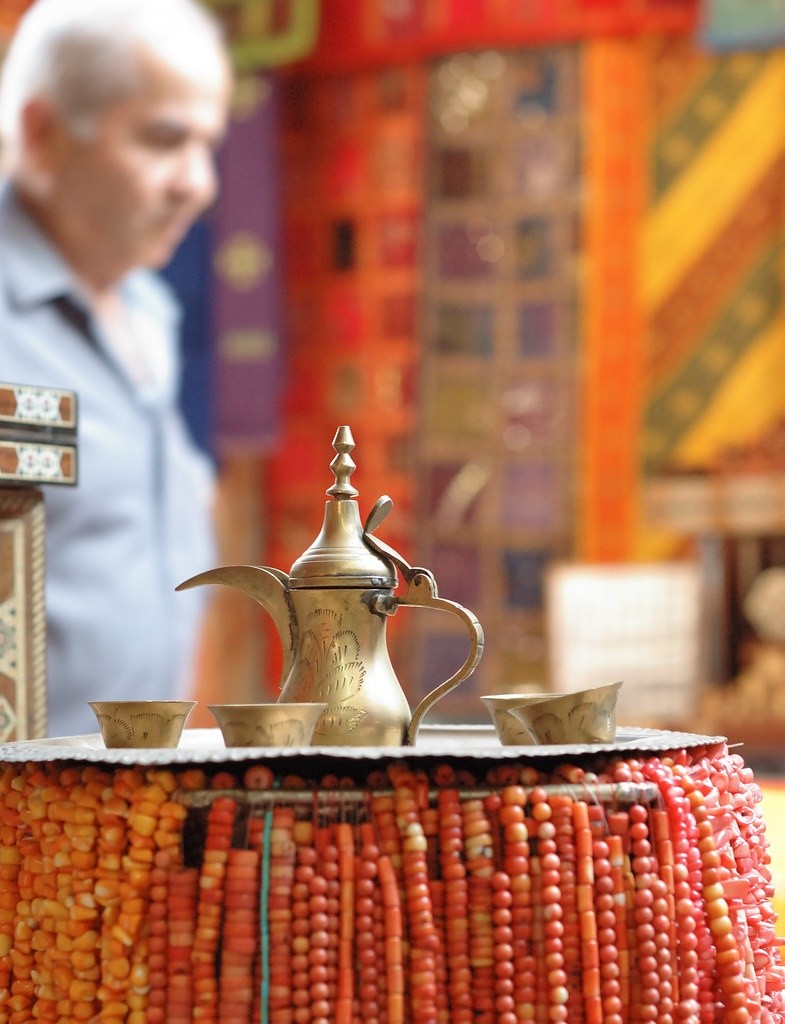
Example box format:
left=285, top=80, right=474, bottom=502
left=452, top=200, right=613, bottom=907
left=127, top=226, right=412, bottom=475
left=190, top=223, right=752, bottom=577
left=171, top=426, right=484, bottom=745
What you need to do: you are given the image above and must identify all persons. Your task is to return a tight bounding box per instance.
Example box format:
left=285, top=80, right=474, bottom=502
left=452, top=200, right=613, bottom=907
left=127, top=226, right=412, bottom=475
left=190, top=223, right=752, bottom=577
left=0, top=0, right=232, bottom=738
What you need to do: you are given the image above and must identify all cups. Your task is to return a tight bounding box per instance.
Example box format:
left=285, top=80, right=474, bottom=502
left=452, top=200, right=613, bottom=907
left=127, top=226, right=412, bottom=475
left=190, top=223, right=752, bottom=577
left=87, top=701, right=198, bottom=749
left=207, top=703, right=330, bottom=748
left=481, top=694, right=563, bottom=746
left=507, top=681, right=624, bottom=745
